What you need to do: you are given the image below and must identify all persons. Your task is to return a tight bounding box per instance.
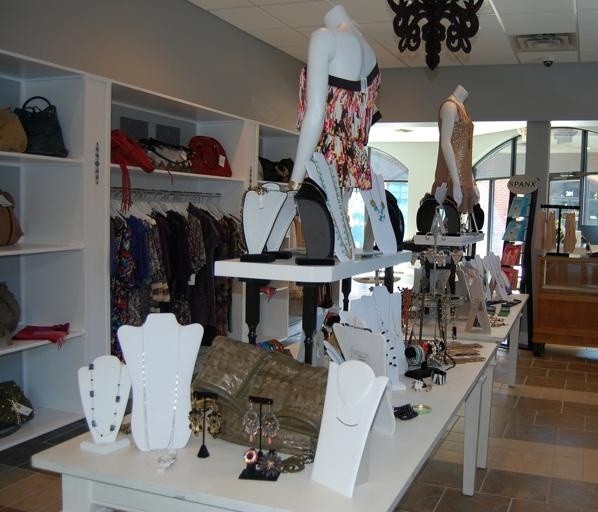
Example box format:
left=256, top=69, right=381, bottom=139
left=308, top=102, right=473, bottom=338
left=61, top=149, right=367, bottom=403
left=430, top=83, right=484, bottom=233
left=289, top=3, right=382, bottom=259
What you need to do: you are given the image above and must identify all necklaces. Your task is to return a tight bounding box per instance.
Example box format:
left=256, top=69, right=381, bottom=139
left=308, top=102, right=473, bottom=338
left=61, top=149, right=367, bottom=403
left=388, top=201, right=403, bottom=232
left=372, top=295, right=392, bottom=332
left=368, top=174, right=386, bottom=223
left=335, top=367, right=374, bottom=427
left=256, top=187, right=281, bottom=210
left=297, top=182, right=327, bottom=203
left=88, top=360, right=124, bottom=438
left=140, top=323, right=182, bottom=452
left=310, top=158, right=353, bottom=260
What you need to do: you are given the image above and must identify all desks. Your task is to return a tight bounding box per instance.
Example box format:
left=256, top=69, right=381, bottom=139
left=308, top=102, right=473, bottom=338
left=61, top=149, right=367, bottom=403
left=401, top=293, right=531, bottom=470
left=30, top=340, right=499, bottom=512
left=212, top=251, right=412, bottom=364
left=414, top=231, right=486, bottom=321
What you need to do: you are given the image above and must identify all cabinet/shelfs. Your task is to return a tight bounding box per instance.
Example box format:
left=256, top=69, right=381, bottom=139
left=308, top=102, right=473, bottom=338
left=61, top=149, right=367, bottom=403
left=257, top=124, right=341, bottom=340
left=532, top=255, right=598, bottom=355
left=111, top=80, right=257, bottom=378
left=503, top=176, right=538, bottom=350
left=1, top=48, right=110, bottom=450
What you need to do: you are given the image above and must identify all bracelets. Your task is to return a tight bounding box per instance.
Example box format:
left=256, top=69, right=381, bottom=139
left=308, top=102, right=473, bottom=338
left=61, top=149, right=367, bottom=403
left=407, top=340, right=442, bottom=366
left=411, top=404, right=434, bottom=414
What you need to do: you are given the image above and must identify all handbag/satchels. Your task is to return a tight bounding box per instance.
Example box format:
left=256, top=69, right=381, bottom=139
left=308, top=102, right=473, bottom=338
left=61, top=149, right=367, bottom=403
left=0, top=380, right=34, bottom=439
left=0, top=280, right=20, bottom=346
left=0, top=191, right=23, bottom=246
left=14, top=95, right=68, bottom=158
left=111, top=129, right=156, bottom=213
left=139, top=137, right=192, bottom=185
left=191, top=335, right=328, bottom=458
left=189, top=136, right=231, bottom=178
left=0, top=107, right=29, bottom=153
left=258, top=156, right=294, bottom=182
